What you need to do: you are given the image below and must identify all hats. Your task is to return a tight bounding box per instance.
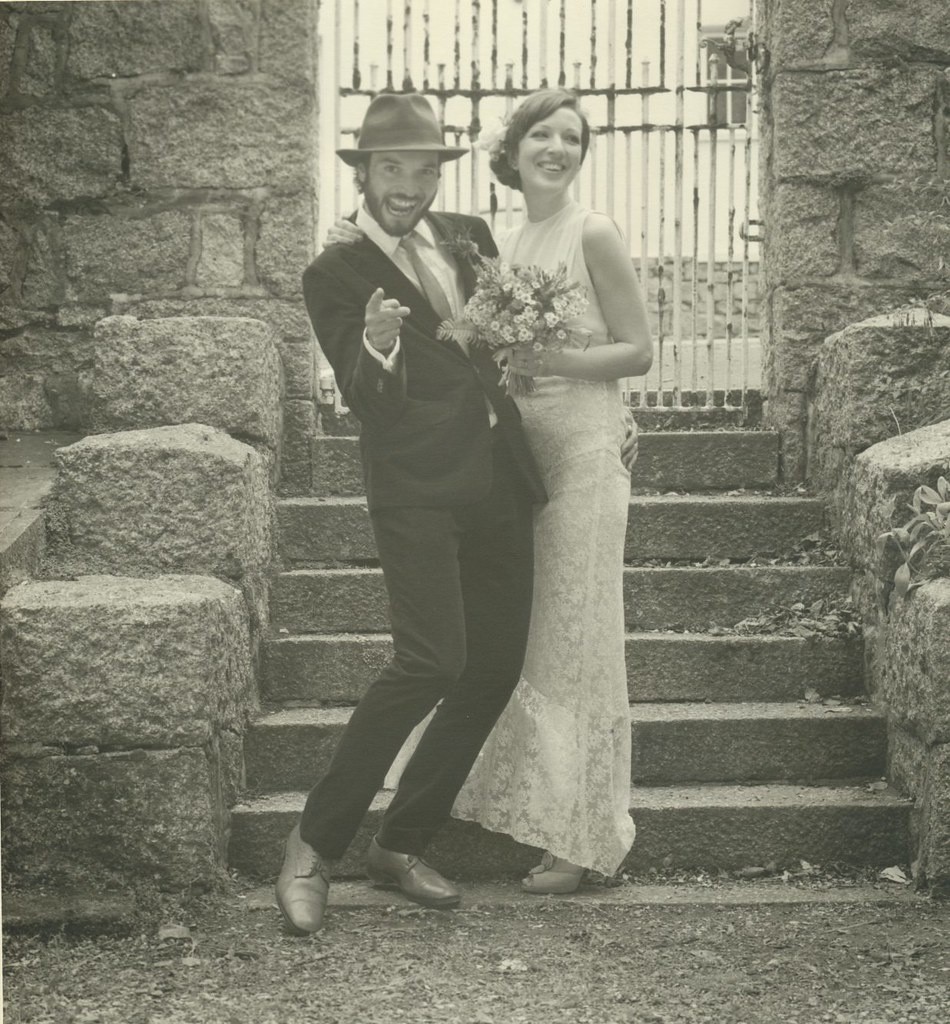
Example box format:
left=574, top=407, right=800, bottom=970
left=334, top=92, right=473, bottom=173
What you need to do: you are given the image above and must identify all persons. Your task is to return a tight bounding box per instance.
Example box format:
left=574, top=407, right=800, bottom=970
left=274, top=93, right=639, bottom=936
left=323, top=89, right=655, bottom=894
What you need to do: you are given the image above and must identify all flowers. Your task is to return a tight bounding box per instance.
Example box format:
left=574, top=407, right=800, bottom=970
left=438, top=227, right=596, bottom=400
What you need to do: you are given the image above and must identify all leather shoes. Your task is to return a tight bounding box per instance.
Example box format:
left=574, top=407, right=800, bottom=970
left=277, top=823, right=330, bottom=937
left=364, top=835, right=460, bottom=907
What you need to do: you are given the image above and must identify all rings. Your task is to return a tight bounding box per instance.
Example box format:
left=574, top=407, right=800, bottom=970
left=521, top=360, right=528, bottom=368
left=380, top=322, right=383, bottom=332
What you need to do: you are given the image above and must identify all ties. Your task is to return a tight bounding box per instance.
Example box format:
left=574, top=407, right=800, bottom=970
left=399, top=231, right=456, bottom=342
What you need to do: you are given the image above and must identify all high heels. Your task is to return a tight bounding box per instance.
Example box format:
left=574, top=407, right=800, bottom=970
left=522, top=853, right=587, bottom=894
